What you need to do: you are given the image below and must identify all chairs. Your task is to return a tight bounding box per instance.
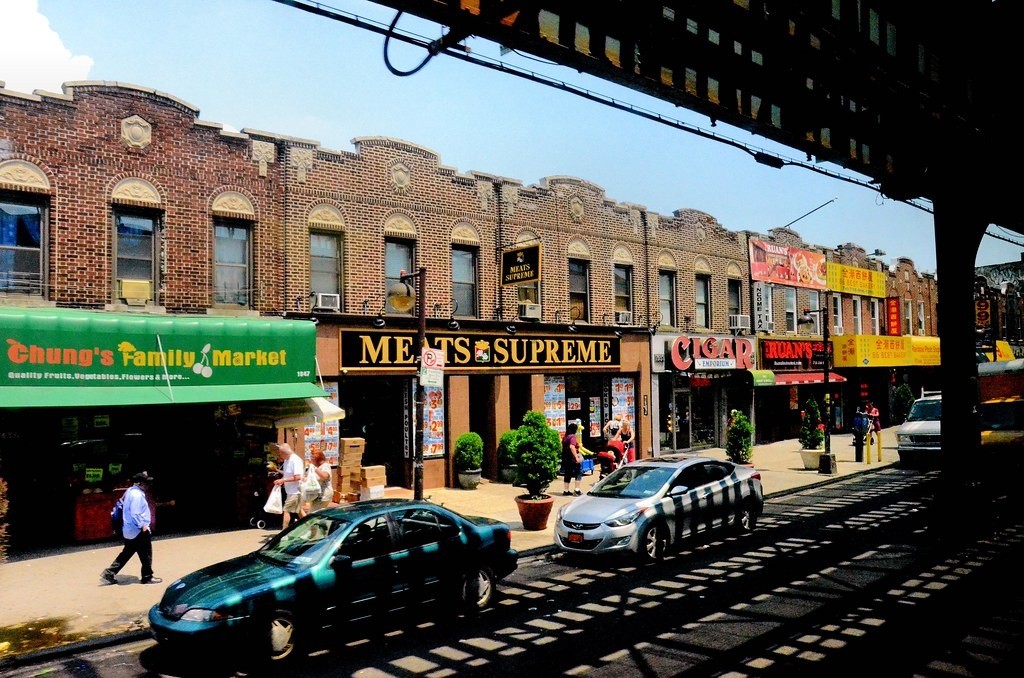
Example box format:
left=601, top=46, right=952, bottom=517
left=649, top=464, right=708, bottom=491
left=333, top=519, right=450, bottom=558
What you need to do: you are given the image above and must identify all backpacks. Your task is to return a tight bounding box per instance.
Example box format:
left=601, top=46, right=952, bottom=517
left=110, top=492, right=124, bottom=543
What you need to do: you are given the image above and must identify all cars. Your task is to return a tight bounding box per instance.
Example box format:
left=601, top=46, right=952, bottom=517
left=148, top=497, right=518, bottom=670
left=552, top=454, right=764, bottom=562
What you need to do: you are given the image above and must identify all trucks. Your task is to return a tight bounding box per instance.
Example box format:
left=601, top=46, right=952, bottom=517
left=977, top=359, right=1024, bottom=461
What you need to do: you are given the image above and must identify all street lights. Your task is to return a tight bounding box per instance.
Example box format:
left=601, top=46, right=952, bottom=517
left=798, top=309, right=838, bottom=476
left=385, top=270, right=424, bottom=500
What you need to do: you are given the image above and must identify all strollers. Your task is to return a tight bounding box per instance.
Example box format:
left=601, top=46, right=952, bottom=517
left=247, top=464, right=285, bottom=528
left=598, top=439, right=632, bottom=483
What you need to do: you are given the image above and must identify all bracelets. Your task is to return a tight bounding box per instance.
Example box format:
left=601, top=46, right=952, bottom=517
left=284, top=478, right=287, bottom=483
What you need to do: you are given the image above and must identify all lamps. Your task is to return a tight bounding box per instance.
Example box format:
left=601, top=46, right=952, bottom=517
left=296, top=291, right=386, bottom=328
left=603, top=313, right=628, bottom=339
left=435, top=298, right=460, bottom=330
left=640, top=311, right=663, bottom=335
left=555, top=306, right=581, bottom=334
left=505, top=303, right=520, bottom=334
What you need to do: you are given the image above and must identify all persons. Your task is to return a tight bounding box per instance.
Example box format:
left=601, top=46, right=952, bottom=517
left=561, top=423, right=586, bottom=497
left=273, top=443, right=334, bottom=540
left=603, top=415, right=636, bottom=471
left=865, top=402, right=881, bottom=439
left=100, top=473, right=163, bottom=584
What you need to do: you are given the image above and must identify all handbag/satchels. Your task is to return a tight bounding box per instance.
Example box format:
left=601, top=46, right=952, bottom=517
left=299, top=464, right=321, bottom=502
left=264, top=485, right=282, bottom=514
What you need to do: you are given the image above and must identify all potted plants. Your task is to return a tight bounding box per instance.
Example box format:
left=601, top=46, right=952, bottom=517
left=890, top=384, right=916, bottom=444
left=499, top=410, right=561, bottom=531
left=726, top=393, right=825, bottom=469
left=453, top=432, right=484, bottom=489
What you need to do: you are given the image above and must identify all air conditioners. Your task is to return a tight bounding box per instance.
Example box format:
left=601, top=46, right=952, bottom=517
left=518, top=303, right=541, bottom=318
left=311, top=293, right=340, bottom=309
left=729, top=315, right=750, bottom=329
left=764, top=322, right=775, bottom=332
left=833, top=326, right=844, bottom=336
left=615, top=311, right=632, bottom=323
left=919, top=329, right=925, bottom=335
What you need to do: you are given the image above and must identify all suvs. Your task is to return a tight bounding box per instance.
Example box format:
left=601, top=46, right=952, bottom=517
left=895, top=389, right=942, bottom=462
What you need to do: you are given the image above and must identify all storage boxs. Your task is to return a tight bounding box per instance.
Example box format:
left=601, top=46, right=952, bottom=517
left=333, top=437, right=386, bottom=504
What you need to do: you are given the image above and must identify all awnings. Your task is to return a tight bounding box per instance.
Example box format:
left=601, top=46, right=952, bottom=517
left=749, top=370, right=847, bottom=386
left=1, top=381, right=332, bottom=407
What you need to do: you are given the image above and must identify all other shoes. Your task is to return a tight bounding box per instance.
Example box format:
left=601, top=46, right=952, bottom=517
left=562, top=490, right=573, bottom=496
left=100, top=572, right=118, bottom=584
left=141, top=576, right=162, bottom=584
left=573, top=490, right=582, bottom=497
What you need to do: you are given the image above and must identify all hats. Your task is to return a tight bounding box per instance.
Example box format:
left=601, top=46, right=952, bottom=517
left=132, top=473, right=153, bottom=483
